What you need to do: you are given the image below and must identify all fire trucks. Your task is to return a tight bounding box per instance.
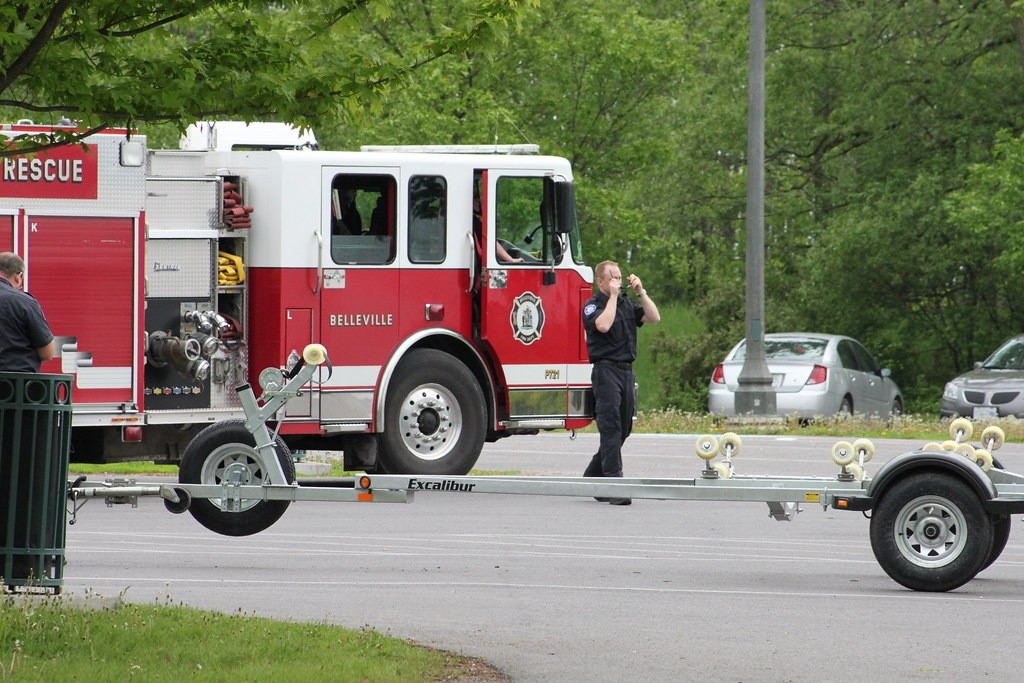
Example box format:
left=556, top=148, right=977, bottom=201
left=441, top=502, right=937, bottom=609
left=0, top=119, right=596, bottom=475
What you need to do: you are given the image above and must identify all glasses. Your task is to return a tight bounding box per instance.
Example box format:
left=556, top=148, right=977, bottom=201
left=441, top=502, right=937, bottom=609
left=609, top=265, right=631, bottom=290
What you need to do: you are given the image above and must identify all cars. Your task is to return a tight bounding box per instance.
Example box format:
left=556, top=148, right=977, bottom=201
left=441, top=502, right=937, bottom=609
left=939, top=334, right=1024, bottom=420
left=708, top=332, right=905, bottom=423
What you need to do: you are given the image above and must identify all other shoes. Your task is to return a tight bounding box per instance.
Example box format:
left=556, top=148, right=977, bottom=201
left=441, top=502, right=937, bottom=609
left=594, top=497, right=631, bottom=505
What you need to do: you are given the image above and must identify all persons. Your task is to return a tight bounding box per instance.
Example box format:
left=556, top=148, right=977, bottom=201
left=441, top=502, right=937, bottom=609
left=473, top=192, right=524, bottom=263
left=582, top=260, right=660, bottom=505
left=0, top=252, right=55, bottom=373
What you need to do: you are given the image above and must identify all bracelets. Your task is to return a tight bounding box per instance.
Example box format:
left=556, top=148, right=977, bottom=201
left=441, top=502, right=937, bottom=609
left=636, top=289, right=646, bottom=297
